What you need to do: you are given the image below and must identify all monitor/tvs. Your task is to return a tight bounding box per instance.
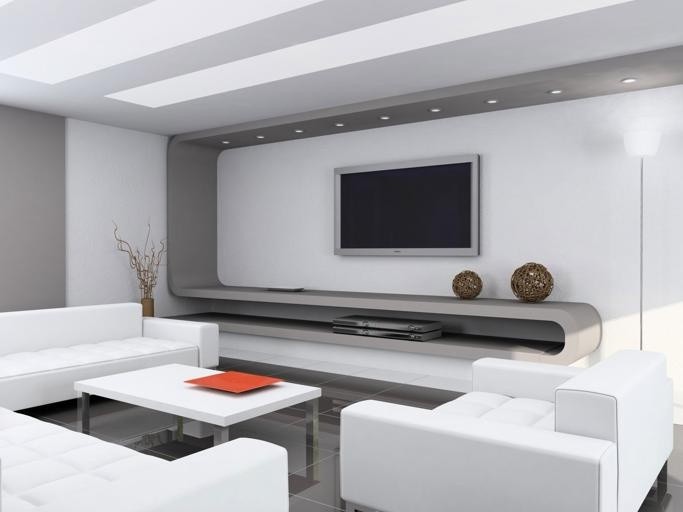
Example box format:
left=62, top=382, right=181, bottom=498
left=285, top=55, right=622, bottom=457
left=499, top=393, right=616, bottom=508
left=333, top=152, right=481, bottom=257
